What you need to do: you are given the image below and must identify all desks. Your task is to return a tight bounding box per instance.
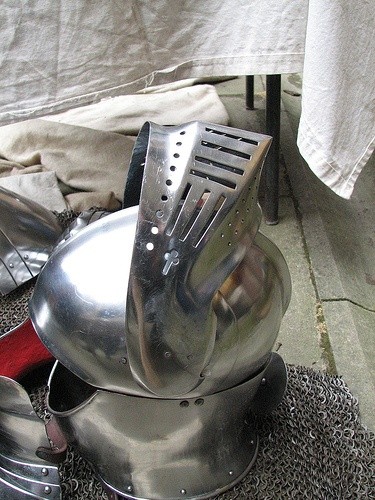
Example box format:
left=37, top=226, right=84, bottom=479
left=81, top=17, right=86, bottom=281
left=0, top=1, right=374, bottom=226
